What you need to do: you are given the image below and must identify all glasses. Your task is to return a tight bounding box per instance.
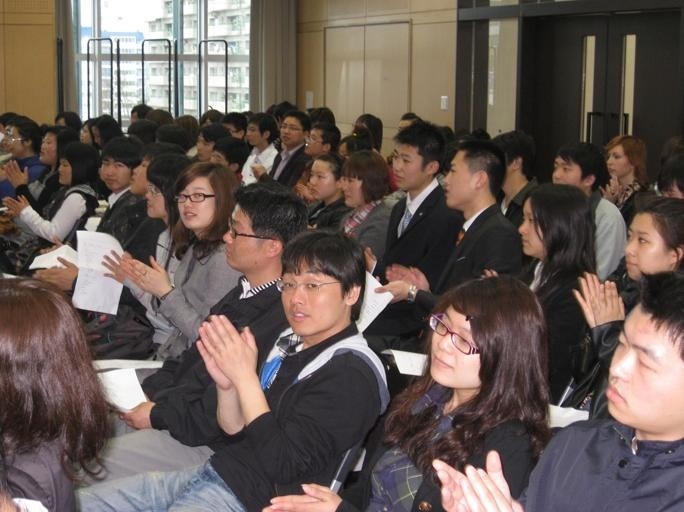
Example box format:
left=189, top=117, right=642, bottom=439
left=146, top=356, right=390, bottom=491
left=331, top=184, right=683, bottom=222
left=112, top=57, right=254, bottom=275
left=427, top=312, right=480, bottom=355
left=146, top=184, right=164, bottom=198
left=174, top=192, right=215, bottom=203
left=276, top=280, right=343, bottom=293
left=227, top=216, right=280, bottom=241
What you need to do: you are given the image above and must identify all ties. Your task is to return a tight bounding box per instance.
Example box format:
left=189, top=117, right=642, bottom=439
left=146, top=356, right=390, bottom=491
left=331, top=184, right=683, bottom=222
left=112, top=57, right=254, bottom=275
left=456, top=229, right=464, bottom=246
left=401, top=209, right=412, bottom=235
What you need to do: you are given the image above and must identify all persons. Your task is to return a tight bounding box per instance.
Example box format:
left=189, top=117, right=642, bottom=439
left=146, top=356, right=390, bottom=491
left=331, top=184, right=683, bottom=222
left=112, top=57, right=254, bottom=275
left=477, top=182, right=596, bottom=406
left=0, top=101, right=683, bottom=312
left=0, top=277, right=113, bottom=512
left=66, top=180, right=310, bottom=490
left=260, top=274, right=551, bottom=512
left=431, top=270, right=684, bottom=512
left=65, top=228, right=393, bottom=512
left=556, top=197, right=684, bottom=418
left=0, top=442, right=52, bottom=512
